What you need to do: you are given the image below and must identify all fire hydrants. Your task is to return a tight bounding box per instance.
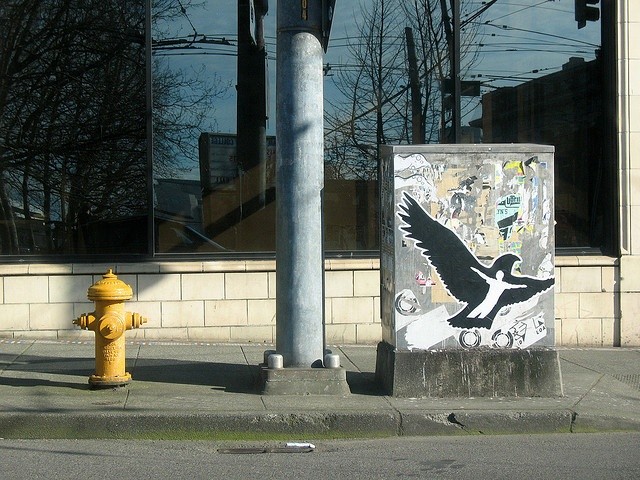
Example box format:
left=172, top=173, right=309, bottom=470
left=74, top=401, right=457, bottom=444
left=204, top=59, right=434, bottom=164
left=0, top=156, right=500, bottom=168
left=72, top=268, right=147, bottom=388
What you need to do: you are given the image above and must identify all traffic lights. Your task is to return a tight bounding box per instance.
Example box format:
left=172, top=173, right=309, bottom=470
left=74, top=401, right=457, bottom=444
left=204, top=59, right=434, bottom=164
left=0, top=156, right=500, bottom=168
left=574, top=1, right=600, bottom=30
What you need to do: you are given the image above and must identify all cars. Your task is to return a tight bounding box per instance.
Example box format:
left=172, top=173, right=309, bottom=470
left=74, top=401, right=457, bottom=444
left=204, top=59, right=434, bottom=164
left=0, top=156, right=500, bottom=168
left=71, top=214, right=236, bottom=252
